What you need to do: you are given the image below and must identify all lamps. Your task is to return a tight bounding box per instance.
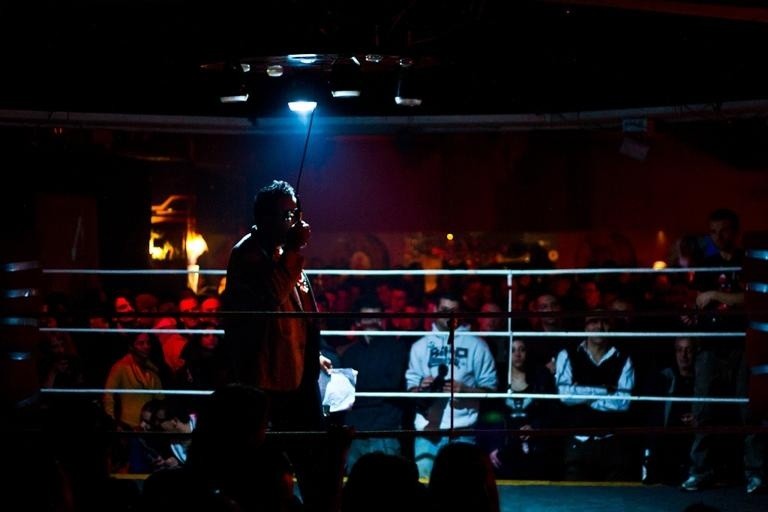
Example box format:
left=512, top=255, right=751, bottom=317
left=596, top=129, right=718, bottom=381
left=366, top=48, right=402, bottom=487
left=218, top=44, right=427, bottom=112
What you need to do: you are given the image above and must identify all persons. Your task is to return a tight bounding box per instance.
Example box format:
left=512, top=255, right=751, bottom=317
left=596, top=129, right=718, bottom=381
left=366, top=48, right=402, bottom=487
left=37, top=179, right=768, bottom=511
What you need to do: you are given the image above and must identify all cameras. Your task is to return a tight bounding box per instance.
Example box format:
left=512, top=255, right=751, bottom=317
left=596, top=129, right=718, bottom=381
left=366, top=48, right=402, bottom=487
left=414, top=364, right=451, bottom=411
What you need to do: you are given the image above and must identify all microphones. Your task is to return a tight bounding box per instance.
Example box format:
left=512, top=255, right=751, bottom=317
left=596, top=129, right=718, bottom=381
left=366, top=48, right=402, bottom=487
left=294, top=196, right=307, bottom=249
